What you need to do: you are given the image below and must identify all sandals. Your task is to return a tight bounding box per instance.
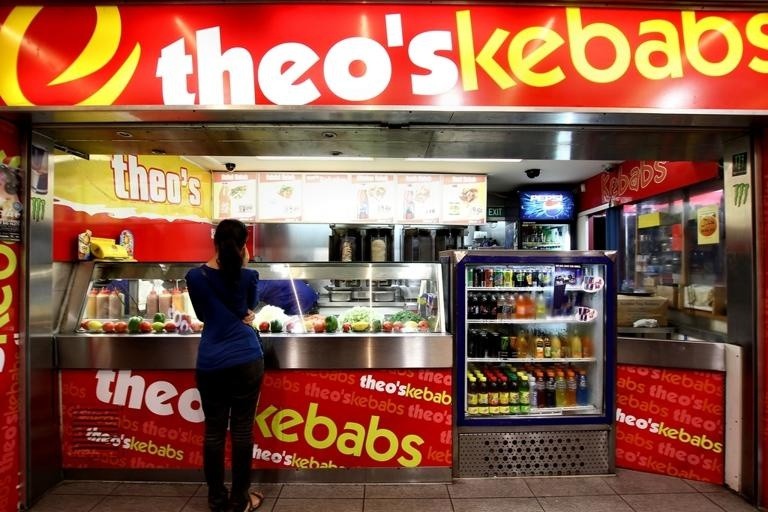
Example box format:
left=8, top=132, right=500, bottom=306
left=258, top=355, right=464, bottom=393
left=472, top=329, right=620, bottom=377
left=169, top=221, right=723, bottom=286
left=245, top=490, right=264, bottom=512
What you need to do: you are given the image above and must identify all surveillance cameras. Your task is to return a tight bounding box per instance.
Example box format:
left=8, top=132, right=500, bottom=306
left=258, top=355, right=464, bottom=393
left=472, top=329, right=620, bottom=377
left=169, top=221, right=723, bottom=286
left=525, top=169, right=541, bottom=178
left=226, top=163, right=236, bottom=171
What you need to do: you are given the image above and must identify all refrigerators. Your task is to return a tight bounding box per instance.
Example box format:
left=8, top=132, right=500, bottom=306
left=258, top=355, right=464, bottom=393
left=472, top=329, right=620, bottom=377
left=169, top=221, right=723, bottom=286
left=446, top=243, right=619, bottom=479
left=502, top=186, right=577, bottom=250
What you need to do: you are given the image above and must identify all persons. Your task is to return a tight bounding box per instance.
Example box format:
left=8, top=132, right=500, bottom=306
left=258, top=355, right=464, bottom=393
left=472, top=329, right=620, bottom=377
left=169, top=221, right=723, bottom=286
left=186, top=219, right=265, bottom=511
left=254, top=280, right=319, bottom=316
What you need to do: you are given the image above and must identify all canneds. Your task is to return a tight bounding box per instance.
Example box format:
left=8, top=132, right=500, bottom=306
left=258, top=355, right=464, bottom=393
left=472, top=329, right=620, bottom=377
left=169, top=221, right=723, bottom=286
left=501, top=336, right=517, bottom=357
left=513, top=269, right=551, bottom=287
left=468, top=268, right=513, bottom=287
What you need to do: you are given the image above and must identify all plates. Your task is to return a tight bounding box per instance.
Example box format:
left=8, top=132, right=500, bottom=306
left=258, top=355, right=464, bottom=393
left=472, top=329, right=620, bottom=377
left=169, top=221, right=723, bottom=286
left=620, top=289, right=655, bottom=295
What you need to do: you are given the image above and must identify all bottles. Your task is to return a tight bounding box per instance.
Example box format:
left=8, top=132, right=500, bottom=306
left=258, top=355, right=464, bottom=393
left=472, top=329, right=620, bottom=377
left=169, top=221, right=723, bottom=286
left=467, top=363, right=590, bottom=406
left=469, top=292, right=547, bottom=318
left=86, top=286, right=191, bottom=319
left=469, top=406, right=532, bottom=416
left=523, top=226, right=560, bottom=243
left=468, top=327, right=590, bottom=358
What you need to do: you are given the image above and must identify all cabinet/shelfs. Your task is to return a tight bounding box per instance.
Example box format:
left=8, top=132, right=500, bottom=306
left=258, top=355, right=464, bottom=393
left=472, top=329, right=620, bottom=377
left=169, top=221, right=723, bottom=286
left=53, top=259, right=455, bottom=484
